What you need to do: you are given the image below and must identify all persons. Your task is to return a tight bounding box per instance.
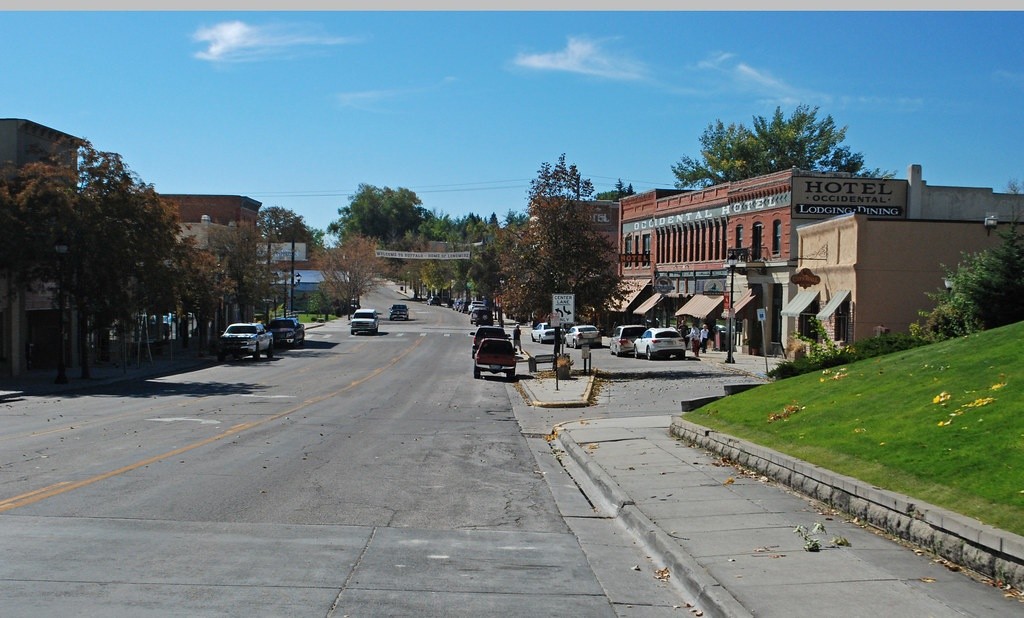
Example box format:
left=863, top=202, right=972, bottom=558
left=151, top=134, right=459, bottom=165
left=699, top=324, right=710, bottom=353
left=533, top=316, right=538, bottom=329
left=690, top=324, right=702, bottom=358
left=678, top=320, right=690, bottom=347
left=513, top=324, right=524, bottom=355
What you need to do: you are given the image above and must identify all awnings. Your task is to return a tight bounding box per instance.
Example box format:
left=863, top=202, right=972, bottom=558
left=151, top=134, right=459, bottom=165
left=634, top=292, right=667, bottom=314
left=721, top=285, right=762, bottom=320
left=815, top=289, right=851, bottom=321
left=603, top=278, right=653, bottom=313
left=674, top=296, right=725, bottom=319
left=780, top=291, right=820, bottom=317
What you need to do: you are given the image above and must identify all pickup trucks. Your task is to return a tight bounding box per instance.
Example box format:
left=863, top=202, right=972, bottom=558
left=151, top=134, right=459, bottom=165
left=219, top=323, right=273, bottom=361
left=267, top=318, right=305, bottom=349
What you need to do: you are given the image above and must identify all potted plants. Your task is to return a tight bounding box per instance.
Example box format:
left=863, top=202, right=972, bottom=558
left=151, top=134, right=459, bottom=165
left=556, top=354, right=570, bottom=379
left=745, top=337, right=763, bottom=355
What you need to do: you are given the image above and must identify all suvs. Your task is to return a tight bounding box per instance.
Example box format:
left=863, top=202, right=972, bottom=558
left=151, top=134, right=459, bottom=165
left=470, top=327, right=512, bottom=359
left=632, top=327, right=686, bottom=360
left=609, top=325, right=648, bottom=357
left=389, top=304, right=410, bottom=320
left=473, top=337, right=516, bottom=381
left=351, top=309, right=378, bottom=335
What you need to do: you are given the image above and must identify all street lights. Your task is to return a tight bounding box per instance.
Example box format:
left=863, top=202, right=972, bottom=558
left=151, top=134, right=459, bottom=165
left=725, top=248, right=739, bottom=363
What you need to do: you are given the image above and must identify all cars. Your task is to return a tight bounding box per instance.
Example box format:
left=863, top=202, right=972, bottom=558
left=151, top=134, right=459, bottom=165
left=427, top=296, right=495, bottom=326
left=529, top=322, right=566, bottom=345
left=564, top=326, right=603, bottom=349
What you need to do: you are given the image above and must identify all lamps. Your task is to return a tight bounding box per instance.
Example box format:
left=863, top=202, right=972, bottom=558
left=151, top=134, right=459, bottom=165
left=984, top=215, right=998, bottom=236
